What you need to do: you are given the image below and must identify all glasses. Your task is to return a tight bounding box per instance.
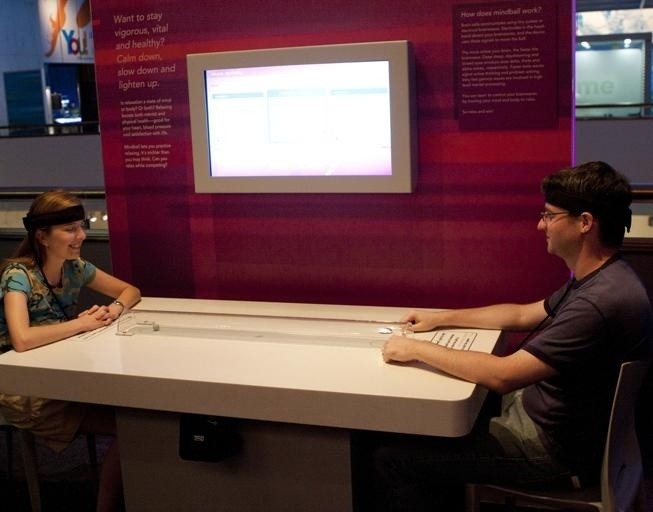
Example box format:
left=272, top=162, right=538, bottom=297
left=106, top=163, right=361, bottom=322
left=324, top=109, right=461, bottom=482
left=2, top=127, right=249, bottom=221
left=542, top=210, right=586, bottom=221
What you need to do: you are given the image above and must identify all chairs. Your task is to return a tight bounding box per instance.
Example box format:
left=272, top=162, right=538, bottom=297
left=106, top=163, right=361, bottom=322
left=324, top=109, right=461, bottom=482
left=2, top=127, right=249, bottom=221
left=456, top=355, right=643, bottom=512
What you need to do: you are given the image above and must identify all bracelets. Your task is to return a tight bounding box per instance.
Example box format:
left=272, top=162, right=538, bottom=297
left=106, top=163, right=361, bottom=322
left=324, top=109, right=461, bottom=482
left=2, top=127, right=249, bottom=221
left=112, top=300, right=124, bottom=310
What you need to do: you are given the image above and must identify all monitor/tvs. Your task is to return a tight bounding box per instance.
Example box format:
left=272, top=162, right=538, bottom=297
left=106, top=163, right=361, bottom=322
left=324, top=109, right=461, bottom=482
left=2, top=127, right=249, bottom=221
left=187, top=40, right=414, bottom=196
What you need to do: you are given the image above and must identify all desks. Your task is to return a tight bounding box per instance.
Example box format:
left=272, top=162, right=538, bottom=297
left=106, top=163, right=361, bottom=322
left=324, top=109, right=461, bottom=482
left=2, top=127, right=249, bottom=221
left=0, top=289, right=503, bottom=511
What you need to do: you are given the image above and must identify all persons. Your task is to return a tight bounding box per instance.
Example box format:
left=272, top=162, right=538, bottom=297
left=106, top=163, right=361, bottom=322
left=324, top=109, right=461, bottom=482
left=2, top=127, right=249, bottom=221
left=0, top=190, right=142, bottom=512
left=368, top=160, right=653, bottom=512
